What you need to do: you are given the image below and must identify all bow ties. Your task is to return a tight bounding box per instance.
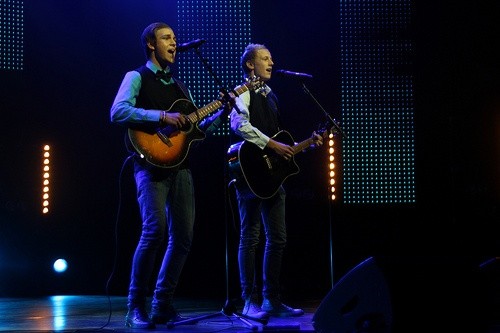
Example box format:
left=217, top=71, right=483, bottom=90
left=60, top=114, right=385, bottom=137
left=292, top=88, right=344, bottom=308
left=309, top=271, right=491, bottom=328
left=155, top=70, right=172, bottom=82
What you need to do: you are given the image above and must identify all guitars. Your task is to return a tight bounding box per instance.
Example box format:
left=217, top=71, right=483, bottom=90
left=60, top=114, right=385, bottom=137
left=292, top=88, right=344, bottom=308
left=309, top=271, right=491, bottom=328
left=126, top=75, right=268, bottom=168
left=226, top=121, right=342, bottom=200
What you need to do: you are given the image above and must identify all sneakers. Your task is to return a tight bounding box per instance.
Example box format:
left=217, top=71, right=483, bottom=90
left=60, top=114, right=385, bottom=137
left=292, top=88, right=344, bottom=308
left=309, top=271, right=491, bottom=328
left=125, top=310, right=153, bottom=329
left=150, top=312, right=196, bottom=324
left=260, top=295, right=304, bottom=316
left=242, top=298, right=269, bottom=321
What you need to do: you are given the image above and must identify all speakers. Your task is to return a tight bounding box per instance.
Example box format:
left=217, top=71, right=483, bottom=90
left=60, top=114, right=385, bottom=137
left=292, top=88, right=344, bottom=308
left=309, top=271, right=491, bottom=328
left=310, top=257, right=500, bottom=333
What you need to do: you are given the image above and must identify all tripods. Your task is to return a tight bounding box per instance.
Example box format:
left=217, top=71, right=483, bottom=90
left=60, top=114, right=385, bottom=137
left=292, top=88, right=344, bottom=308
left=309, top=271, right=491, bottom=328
left=164, top=48, right=261, bottom=332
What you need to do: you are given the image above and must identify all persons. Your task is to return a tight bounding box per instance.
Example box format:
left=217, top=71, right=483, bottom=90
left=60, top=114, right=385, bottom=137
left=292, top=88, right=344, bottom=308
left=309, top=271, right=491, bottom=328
left=110, top=23, right=236, bottom=329
left=230, top=44, right=323, bottom=321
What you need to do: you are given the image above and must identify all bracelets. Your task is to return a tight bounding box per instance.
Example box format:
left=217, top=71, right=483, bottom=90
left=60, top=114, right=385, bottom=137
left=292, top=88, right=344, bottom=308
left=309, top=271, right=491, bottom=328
left=162, top=111, right=166, bottom=121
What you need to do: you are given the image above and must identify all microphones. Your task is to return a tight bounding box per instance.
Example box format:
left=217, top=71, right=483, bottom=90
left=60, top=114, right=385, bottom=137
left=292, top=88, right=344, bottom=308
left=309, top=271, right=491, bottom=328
left=275, top=69, right=313, bottom=81
left=177, top=39, right=206, bottom=52
